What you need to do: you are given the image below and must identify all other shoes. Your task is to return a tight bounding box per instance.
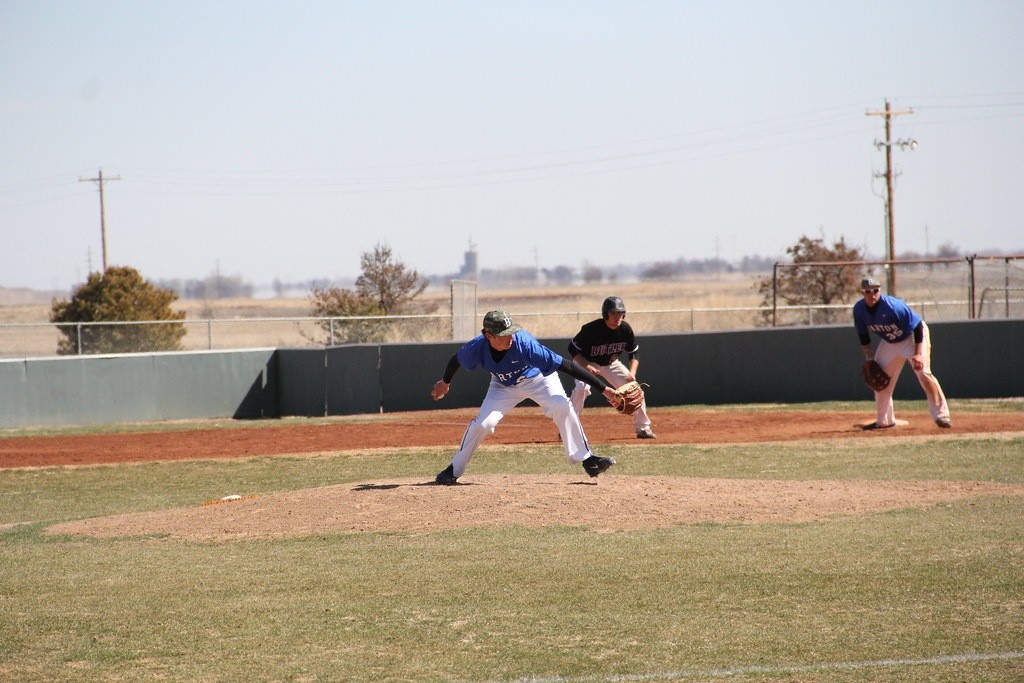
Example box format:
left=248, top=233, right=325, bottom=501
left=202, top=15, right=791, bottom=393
left=936, top=418, right=952, bottom=428
left=862, top=422, right=895, bottom=431
left=637, top=430, right=656, bottom=439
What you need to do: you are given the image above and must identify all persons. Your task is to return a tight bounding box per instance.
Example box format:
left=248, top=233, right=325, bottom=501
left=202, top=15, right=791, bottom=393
left=558, top=297, right=656, bottom=439
left=431, top=311, right=645, bottom=483
left=853, top=277, right=952, bottom=431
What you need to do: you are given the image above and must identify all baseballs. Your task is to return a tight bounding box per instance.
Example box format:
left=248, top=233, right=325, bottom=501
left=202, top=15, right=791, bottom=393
left=433, top=391, right=445, bottom=399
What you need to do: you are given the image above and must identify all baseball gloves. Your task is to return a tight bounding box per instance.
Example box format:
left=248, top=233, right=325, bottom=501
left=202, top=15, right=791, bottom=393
left=863, top=360, right=890, bottom=393
left=608, top=381, right=643, bottom=415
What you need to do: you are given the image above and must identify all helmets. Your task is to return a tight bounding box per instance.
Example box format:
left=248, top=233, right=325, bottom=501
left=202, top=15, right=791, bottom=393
left=602, top=296, right=626, bottom=320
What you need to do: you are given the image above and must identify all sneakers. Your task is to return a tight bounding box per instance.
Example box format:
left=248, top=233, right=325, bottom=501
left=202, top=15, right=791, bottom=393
left=582, top=455, right=617, bottom=478
left=436, top=465, right=456, bottom=485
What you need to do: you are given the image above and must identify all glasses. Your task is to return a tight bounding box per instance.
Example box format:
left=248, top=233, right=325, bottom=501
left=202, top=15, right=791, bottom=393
left=862, top=287, right=880, bottom=295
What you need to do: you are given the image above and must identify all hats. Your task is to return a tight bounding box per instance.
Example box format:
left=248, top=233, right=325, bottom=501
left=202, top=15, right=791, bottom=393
left=862, top=278, right=881, bottom=288
left=483, top=310, right=522, bottom=337
